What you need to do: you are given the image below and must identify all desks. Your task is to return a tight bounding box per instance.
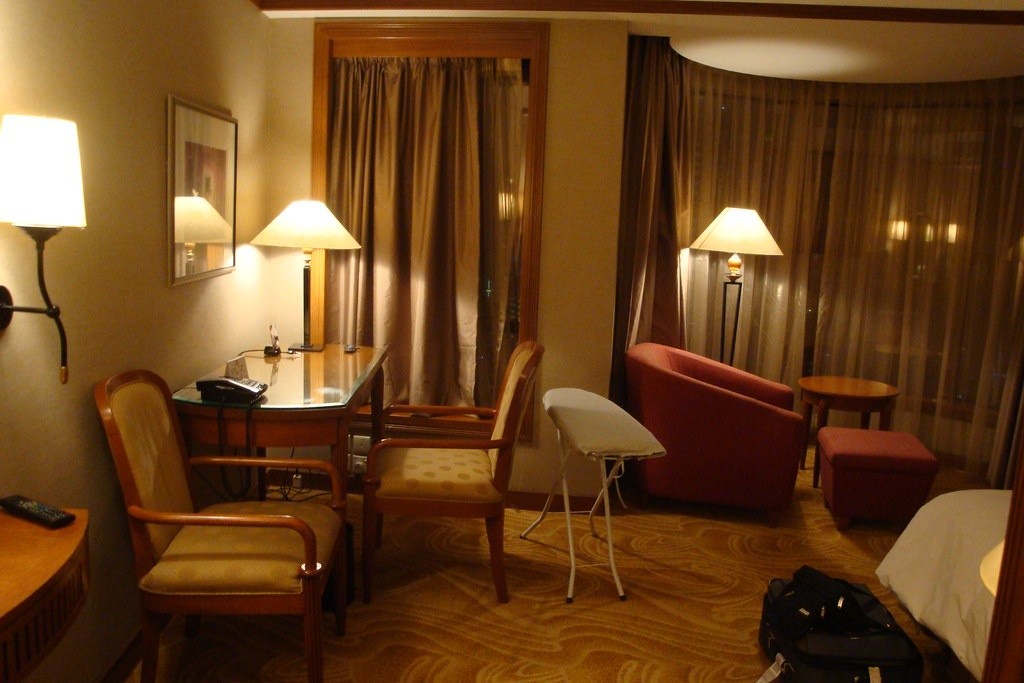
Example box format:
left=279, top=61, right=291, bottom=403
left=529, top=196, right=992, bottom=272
left=0, top=507, right=91, bottom=683
left=797, top=376, right=899, bottom=487
left=519, top=387, right=666, bottom=603
left=172, top=344, right=388, bottom=603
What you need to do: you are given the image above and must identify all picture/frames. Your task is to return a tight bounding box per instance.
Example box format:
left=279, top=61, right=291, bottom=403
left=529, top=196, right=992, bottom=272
left=167, top=94, right=238, bottom=287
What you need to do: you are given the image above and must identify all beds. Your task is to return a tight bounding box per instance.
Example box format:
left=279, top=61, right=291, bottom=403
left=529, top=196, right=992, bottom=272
left=876, top=490, right=1013, bottom=683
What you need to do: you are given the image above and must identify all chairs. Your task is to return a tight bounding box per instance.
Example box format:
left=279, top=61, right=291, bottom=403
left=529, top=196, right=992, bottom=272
left=95, top=370, right=348, bottom=683
left=362, top=340, right=545, bottom=605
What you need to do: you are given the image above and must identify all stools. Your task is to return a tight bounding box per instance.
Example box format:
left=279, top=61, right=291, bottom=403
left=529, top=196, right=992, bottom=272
left=818, top=426, right=940, bottom=531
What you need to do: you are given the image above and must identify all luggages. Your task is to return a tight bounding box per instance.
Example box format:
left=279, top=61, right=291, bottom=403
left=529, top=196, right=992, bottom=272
left=759, top=578, right=924, bottom=683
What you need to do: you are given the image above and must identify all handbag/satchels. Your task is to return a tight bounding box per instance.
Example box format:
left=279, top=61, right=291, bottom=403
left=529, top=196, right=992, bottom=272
left=775, top=564, right=864, bottom=642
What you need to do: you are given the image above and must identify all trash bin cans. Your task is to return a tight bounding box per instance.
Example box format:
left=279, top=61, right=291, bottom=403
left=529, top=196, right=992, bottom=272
left=322, top=522, right=355, bottom=612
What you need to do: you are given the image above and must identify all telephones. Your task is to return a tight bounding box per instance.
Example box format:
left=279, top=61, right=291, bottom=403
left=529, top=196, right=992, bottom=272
left=195, top=373, right=269, bottom=404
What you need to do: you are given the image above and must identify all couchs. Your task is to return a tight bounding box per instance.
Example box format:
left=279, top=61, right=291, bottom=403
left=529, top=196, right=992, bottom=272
left=625, top=342, right=807, bottom=527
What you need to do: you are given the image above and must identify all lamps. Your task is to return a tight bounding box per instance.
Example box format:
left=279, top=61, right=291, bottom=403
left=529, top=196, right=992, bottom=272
left=691, top=207, right=784, bottom=366
left=0, top=115, right=88, bottom=385
left=249, top=200, right=362, bottom=352
left=174, top=196, right=232, bottom=276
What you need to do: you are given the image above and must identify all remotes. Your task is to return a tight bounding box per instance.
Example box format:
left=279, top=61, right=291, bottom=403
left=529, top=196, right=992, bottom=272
left=0, top=495, right=76, bottom=529
